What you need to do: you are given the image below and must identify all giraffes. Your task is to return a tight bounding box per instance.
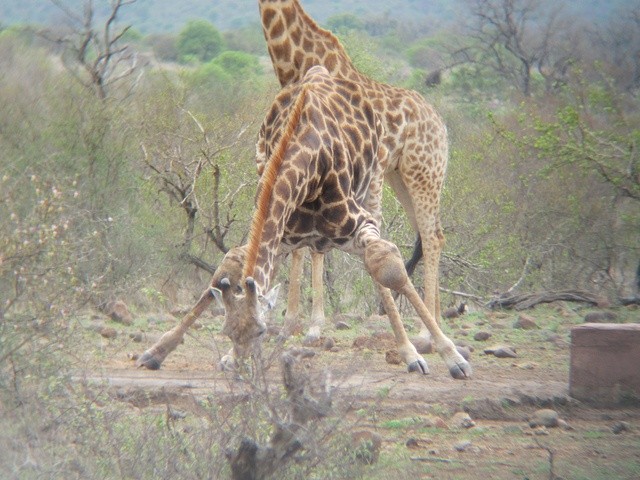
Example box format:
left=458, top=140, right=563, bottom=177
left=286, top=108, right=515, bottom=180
left=134, top=64, right=473, bottom=382
left=258, top=0, right=450, bottom=338
